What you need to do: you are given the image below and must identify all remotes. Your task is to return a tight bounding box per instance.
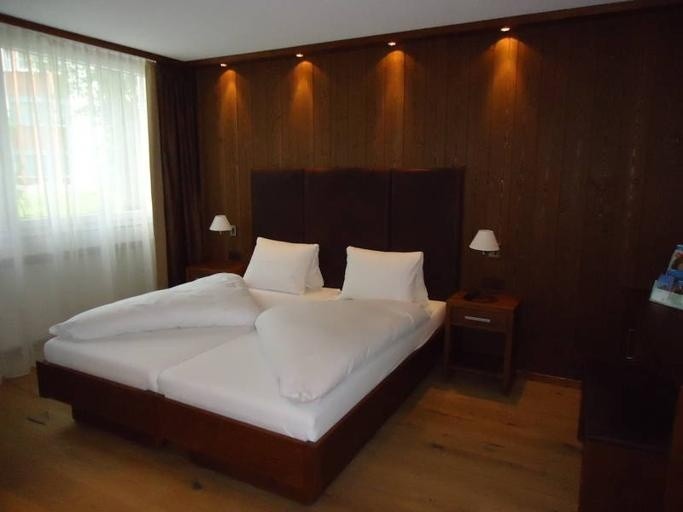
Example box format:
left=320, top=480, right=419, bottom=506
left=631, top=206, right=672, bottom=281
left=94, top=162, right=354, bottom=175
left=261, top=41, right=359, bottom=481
left=464, top=288, right=479, bottom=300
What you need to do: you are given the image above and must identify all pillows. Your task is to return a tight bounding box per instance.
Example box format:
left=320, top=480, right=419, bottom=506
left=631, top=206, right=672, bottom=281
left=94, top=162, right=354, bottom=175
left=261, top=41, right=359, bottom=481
left=243, top=237, right=429, bottom=307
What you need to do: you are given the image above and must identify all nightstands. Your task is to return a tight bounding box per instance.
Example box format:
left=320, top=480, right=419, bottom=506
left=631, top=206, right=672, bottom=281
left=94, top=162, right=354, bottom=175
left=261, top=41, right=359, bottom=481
left=186, top=261, right=247, bottom=281
left=442, top=289, right=522, bottom=392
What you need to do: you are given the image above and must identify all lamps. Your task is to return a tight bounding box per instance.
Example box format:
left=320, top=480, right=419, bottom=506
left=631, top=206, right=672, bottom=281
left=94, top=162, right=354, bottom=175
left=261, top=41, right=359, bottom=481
left=209, top=214, right=236, bottom=237
left=469, top=229, right=501, bottom=258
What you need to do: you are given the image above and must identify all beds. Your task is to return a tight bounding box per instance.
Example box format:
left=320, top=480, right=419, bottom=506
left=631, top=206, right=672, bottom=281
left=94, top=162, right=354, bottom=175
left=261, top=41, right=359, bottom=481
left=36, top=169, right=465, bottom=506
